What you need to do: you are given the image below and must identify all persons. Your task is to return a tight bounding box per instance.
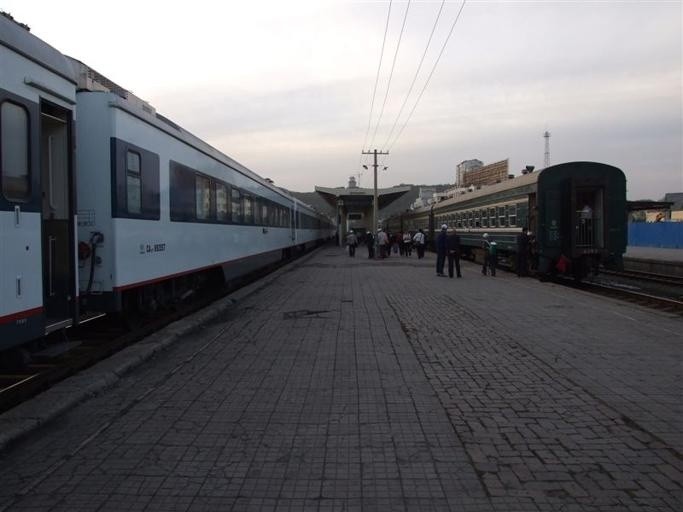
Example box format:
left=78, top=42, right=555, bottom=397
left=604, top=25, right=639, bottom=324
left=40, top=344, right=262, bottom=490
left=485, top=241, right=498, bottom=277
left=580, top=200, right=594, bottom=246
left=445, top=227, right=462, bottom=278
left=479, top=232, right=489, bottom=276
left=363, top=227, right=429, bottom=259
left=345, top=230, right=358, bottom=257
left=515, top=227, right=531, bottom=279
left=433, top=223, right=448, bottom=277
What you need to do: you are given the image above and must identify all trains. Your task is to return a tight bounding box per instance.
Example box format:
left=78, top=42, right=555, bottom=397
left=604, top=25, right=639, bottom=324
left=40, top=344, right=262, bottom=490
left=0, top=13, right=337, bottom=369
left=382, top=161, right=628, bottom=277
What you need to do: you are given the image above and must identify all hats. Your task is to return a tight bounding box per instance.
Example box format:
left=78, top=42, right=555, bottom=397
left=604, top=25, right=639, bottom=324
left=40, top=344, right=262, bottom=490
left=489, top=241, right=496, bottom=246
left=481, top=233, right=488, bottom=239
left=439, top=223, right=448, bottom=229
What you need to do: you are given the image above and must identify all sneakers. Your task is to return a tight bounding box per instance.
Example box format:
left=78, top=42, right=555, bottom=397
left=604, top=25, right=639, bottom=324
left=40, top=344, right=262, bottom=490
left=435, top=272, right=448, bottom=277
